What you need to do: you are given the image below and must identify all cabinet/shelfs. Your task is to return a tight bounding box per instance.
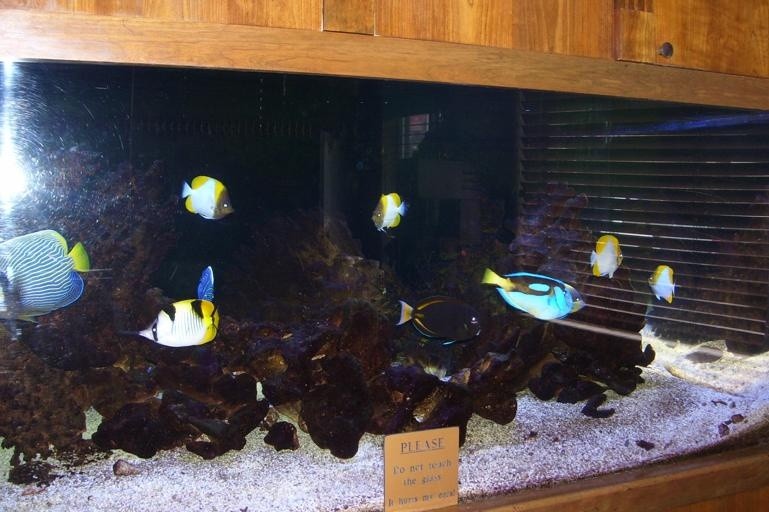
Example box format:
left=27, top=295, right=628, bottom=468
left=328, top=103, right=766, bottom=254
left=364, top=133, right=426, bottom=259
left=1, top=1, right=769, bottom=81
